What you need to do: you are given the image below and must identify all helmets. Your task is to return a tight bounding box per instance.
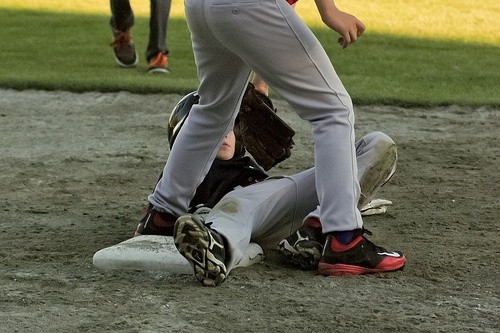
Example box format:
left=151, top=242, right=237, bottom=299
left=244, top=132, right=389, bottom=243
left=168, top=90, right=199, bottom=151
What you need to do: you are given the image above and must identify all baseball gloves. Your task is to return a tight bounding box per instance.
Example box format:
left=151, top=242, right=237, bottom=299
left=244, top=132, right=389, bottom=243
left=233, top=83, right=297, bottom=171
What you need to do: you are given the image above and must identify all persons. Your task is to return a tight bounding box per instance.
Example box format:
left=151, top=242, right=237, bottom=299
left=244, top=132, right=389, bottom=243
left=110, top=0, right=171, bottom=74
left=134, top=0, right=407, bottom=276
left=168, top=71, right=398, bottom=285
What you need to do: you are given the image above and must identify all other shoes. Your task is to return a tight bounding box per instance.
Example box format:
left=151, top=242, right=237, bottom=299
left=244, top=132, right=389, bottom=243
left=148, top=52, right=170, bottom=73
left=110, top=28, right=139, bottom=67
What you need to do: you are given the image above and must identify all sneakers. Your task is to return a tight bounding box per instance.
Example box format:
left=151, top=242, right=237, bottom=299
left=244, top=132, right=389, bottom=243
left=278, top=225, right=324, bottom=270
left=134, top=208, right=175, bottom=236
left=317, top=233, right=407, bottom=275
left=173, top=210, right=228, bottom=287
